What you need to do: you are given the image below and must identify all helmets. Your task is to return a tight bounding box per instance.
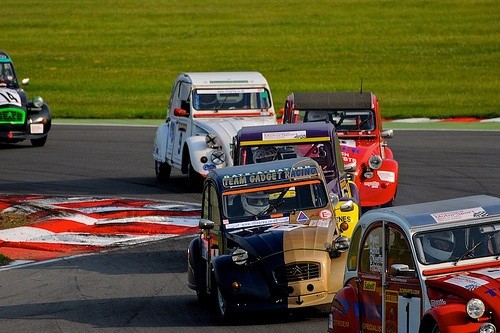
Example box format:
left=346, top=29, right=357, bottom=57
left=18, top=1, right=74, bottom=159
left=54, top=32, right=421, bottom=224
left=307, top=110, right=329, bottom=122
left=423, top=230, right=455, bottom=262
left=240, top=193, right=269, bottom=214
left=253, top=144, right=280, bottom=164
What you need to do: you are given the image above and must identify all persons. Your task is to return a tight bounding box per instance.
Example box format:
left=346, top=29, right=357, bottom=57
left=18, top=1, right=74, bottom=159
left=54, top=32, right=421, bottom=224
left=241, top=192, right=277, bottom=217
left=410, top=230, right=456, bottom=271
left=253, top=148, right=278, bottom=163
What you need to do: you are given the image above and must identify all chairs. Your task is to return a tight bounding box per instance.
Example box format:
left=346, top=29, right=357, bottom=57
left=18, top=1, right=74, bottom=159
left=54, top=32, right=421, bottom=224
left=231, top=195, right=244, bottom=217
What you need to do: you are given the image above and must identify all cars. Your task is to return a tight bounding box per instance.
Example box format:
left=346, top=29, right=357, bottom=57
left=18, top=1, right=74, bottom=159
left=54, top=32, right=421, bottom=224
left=230, top=122, right=362, bottom=246
left=153, top=71, right=278, bottom=186
left=186, top=157, right=352, bottom=317
left=326, top=194, right=500, bottom=333
left=281, top=90, right=398, bottom=212
left=0, top=51, right=52, bottom=146
